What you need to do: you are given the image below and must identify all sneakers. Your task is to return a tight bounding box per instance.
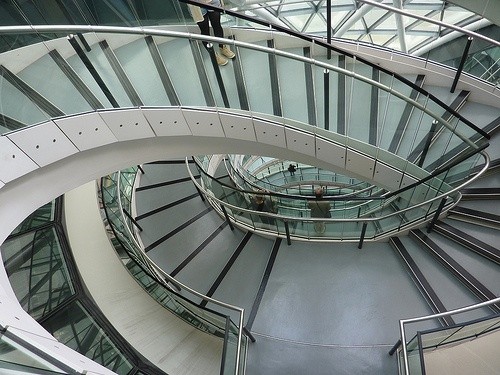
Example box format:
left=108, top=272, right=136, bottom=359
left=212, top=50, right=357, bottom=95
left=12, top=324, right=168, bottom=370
left=219, top=44, right=236, bottom=59
left=214, top=51, right=228, bottom=66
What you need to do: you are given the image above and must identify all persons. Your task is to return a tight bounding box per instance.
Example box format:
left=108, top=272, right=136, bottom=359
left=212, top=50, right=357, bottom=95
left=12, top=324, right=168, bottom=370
left=288, top=163, right=296, bottom=176
left=186, top=0, right=236, bottom=66
left=307, top=187, right=331, bottom=235
left=265, top=198, right=279, bottom=223
left=249, top=189, right=276, bottom=226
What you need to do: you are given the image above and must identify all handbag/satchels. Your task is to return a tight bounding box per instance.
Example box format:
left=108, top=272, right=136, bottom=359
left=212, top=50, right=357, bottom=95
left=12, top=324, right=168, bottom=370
left=269, top=205, right=279, bottom=214
left=324, top=211, right=331, bottom=218
